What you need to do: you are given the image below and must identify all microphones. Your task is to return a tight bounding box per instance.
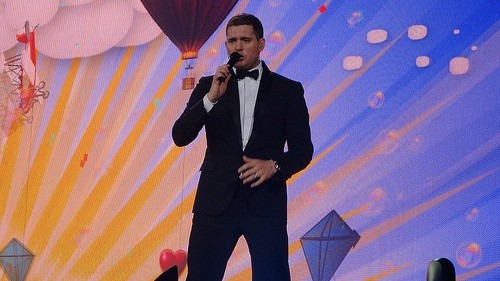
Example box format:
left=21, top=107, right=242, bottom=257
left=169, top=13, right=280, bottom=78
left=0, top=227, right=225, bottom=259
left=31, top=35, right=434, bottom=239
left=218, top=52, right=241, bottom=82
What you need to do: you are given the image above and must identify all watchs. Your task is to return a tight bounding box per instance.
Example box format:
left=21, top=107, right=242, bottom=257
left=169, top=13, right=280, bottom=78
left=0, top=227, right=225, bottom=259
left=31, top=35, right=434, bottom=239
left=272, top=159, right=280, bottom=171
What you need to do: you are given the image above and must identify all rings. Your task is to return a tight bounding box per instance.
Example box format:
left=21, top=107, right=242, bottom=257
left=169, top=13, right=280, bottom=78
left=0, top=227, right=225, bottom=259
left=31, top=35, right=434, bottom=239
left=255, top=173, right=259, bottom=178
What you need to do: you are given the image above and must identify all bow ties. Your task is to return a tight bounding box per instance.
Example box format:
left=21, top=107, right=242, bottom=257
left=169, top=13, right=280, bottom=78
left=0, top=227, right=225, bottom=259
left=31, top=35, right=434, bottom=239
left=236, top=68, right=260, bottom=81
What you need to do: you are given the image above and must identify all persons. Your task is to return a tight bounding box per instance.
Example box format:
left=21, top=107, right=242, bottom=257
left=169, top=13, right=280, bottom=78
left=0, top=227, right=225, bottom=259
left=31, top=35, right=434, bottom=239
left=172, top=13, right=314, bottom=281
